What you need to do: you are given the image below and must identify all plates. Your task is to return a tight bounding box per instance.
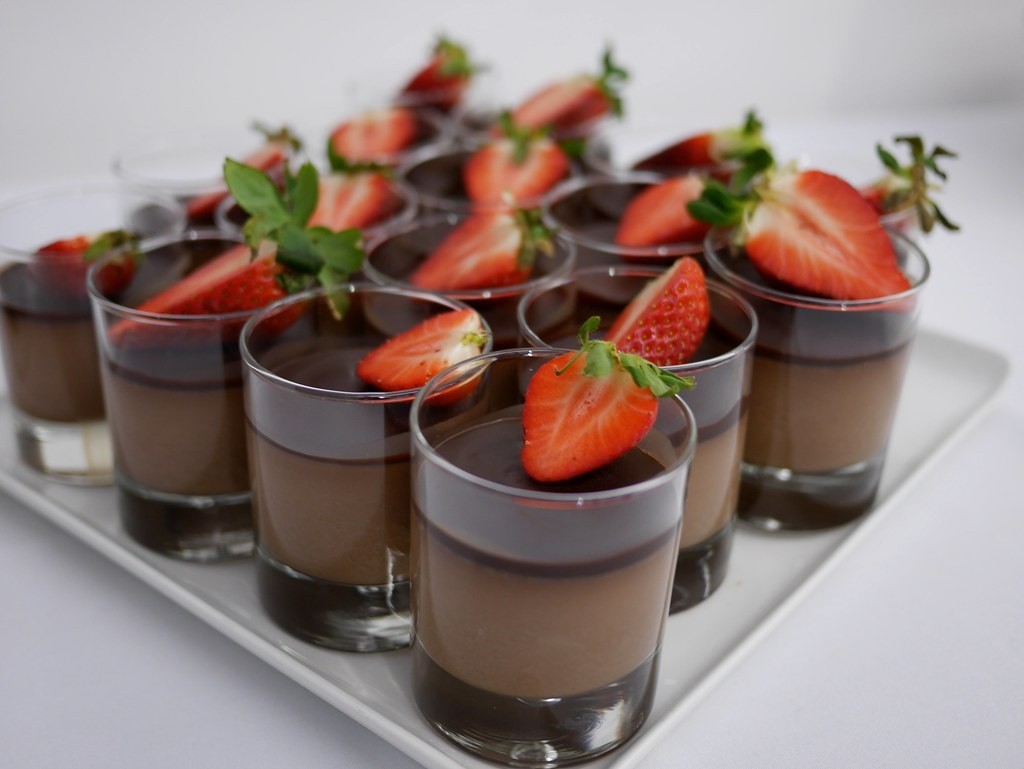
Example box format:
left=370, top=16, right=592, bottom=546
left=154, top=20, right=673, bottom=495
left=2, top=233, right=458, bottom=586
left=0, top=324, right=1009, bottom=769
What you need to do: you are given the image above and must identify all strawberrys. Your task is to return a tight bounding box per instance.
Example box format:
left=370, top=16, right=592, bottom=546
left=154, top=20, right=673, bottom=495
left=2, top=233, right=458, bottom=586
left=37, top=30, right=959, bottom=484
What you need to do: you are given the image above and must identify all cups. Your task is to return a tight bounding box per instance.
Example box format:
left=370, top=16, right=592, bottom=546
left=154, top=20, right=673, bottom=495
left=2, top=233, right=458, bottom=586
left=0, top=182, right=186, bottom=486
left=410, top=348, right=697, bottom=769
left=113, top=115, right=305, bottom=232
left=86, top=232, right=318, bottom=564
left=239, top=286, right=493, bottom=652
left=518, top=266, right=759, bottom=617
left=360, top=214, right=577, bottom=352
left=306, top=108, right=455, bottom=170
left=542, top=175, right=705, bottom=279
left=398, top=142, right=583, bottom=220
left=774, top=137, right=963, bottom=233
left=706, top=225, right=930, bottom=532
left=459, top=89, right=628, bottom=175
left=583, top=116, right=780, bottom=177
left=216, top=170, right=419, bottom=282
